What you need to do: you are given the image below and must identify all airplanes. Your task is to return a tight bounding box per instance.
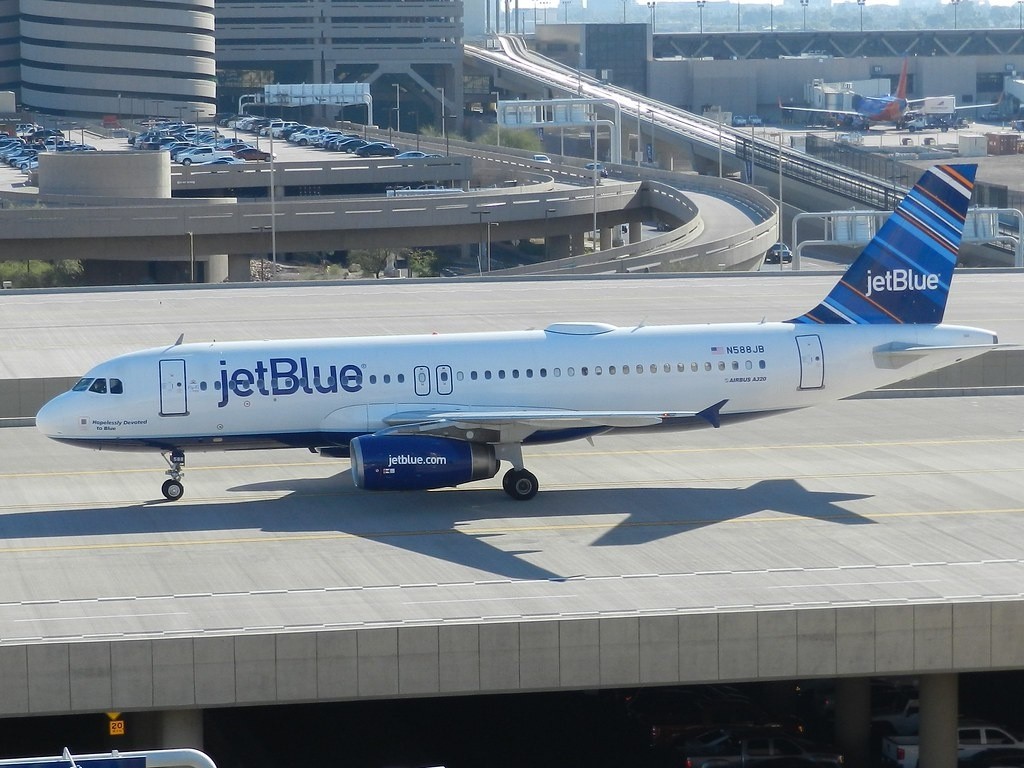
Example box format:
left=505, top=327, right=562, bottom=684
left=37, top=161, right=1021, bottom=500
left=777, top=53, right=1004, bottom=133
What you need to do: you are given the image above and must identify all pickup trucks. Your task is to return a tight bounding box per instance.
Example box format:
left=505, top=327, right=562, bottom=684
left=733, top=116, right=746, bottom=127
left=749, top=115, right=761, bottom=127
left=177, top=147, right=233, bottom=166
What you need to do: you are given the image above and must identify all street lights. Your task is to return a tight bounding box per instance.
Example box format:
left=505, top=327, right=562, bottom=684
left=50, top=119, right=63, bottom=145
left=647, top=110, right=654, bottom=165
left=17, top=104, right=50, bottom=124
left=230, top=120, right=244, bottom=157
left=150, top=100, right=165, bottom=125
left=74, top=126, right=91, bottom=150
left=697, top=0, right=706, bottom=33
left=392, top=83, right=400, bottom=132
left=857, top=0, right=865, bottom=30
left=951, top=0, right=959, bottom=28
left=173, top=107, right=189, bottom=134
left=117, top=94, right=146, bottom=112
left=186, top=231, right=193, bottom=282
left=437, top=87, right=444, bottom=140
left=62, top=122, right=77, bottom=151
left=470, top=210, right=490, bottom=272
left=209, top=113, right=224, bottom=150
left=545, top=209, right=556, bottom=260
left=251, top=122, right=264, bottom=162
left=192, top=110, right=205, bottom=144
left=647, top=2, right=655, bottom=25
left=491, top=91, right=500, bottom=148
left=251, top=226, right=272, bottom=281
left=265, top=127, right=277, bottom=261
left=800, top=0, right=809, bottom=32
left=770, top=131, right=784, bottom=270
left=576, top=51, right=584, bottom=96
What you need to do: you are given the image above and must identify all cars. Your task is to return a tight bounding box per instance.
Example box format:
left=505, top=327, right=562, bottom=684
left=373, top=141, right=394, bottom=147
left=765, top=243, right=793, bottom=264
left=423, top=154, right=444, bottom=158
left=0, top=123, right=99, bottom=175
left=354, top=144, right=400, bottom=158
left=530, top=155, right=551, bottom=169
left=235, top=148, right=277, bottom=162
left=204, top=156, right=246, bottom=165
left=584, top=163, right=608, bottom=179
left=395, top=151, right=426, bottom=167
left=656, top=218, right=673, bottom=232
left=128, top=112, right=360, bottom=151
left=338, top=139, right=371, bottom=154
left=621, top=224, right=628, bottom=234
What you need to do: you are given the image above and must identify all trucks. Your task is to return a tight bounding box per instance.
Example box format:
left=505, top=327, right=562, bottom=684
left=908, top=117, right=970, bottom=133
